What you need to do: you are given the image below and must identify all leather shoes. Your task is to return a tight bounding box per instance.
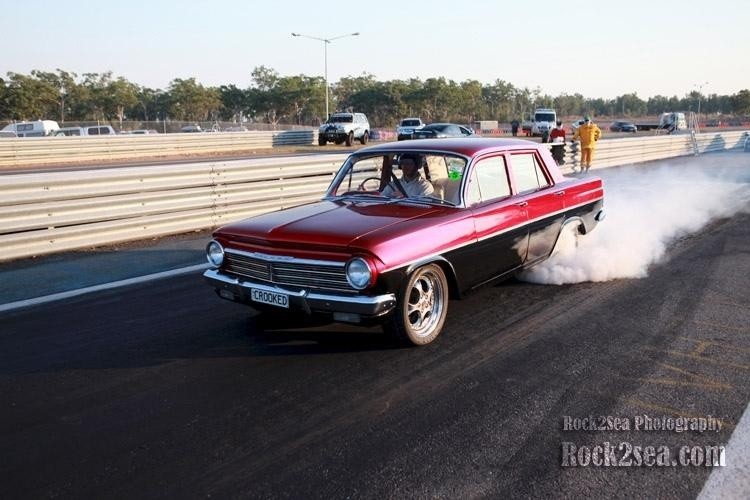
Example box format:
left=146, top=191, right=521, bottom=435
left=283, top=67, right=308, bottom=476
left=594, top=139, right=597, bottom=141
left=571, top=140, right=574, bottom=143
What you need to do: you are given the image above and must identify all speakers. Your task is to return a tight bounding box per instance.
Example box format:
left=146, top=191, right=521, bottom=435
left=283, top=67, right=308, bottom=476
left=586, top=167, right=591, bottom=174
left=580, top=167, right=584, bottom=174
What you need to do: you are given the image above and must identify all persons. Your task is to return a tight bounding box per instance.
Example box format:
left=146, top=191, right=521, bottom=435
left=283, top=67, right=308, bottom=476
left=511, top=118, right=519, bottom=136
left=542, top=127, right=549, bottom=142
left=380, top=155, right=434, bottom=199
left=551, top=119, right=565, bottom=145
left=572, top=115, right=601, bottom=174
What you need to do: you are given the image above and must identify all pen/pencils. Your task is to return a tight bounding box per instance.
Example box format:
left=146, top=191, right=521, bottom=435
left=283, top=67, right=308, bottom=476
left=661, top=112, right=687, bottom=131
left=531, top=109, right=557, bottom=137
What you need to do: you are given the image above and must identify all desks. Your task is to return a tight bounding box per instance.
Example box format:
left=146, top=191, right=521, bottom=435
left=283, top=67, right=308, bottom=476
left=694, top=81, right=710, bottom=122
left=292, top=30, right=361, bottom=123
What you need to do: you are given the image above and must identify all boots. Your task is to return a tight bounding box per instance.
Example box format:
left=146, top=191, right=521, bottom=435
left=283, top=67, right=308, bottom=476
left=396, top=118, right=425, bottom=140
left=317, top=111, right=370, bottom=146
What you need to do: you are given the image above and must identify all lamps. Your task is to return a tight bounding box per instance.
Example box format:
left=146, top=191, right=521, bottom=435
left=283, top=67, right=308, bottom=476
left=431, top=172, right=465, bottom=206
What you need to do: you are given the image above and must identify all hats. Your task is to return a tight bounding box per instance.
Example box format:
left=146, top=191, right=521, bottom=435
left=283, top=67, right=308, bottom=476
left=413, top=122, right=475, bottom=141
left=610, top=121, right=637, bottom=133
left=1, top=118, right=248, bottom=143
left=707, top=118, right=719, bottom=127
left=571, top=120, right=585, bottom=134
left=200, top=136, right=605, bottom=350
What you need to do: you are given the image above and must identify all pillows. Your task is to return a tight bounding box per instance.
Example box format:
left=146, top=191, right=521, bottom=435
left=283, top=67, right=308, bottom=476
left=584, top=116, right=590, bottom=125
left=556, top=119, right=562, bottom=130
